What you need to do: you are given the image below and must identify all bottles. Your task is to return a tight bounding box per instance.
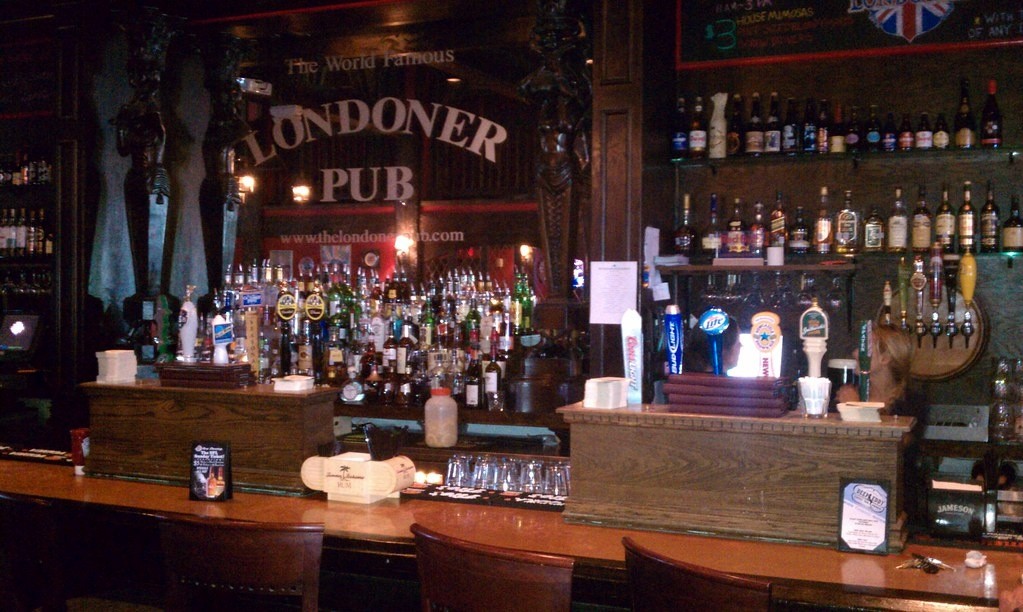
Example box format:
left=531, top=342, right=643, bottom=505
left=812, top=187, right=834, bottom=254
left=979, top=79, right=1002, bottom=149
left=911, top=184, right=932, bottom=252
left=748, top=200, right=769, bottom=254
left=915, top=111, right=932, bottom=150
left=884, top=112, right=914, bottom=152
left=216, top=466, right=226, bottom=498
left=935, top=184, right=955, bottom=254
left=0, top=263, right=53, bottom=314
left=0, top=207, right=54, bottom=258
left=845, top=104, right=883, bottom=154
left=703, top=193, right=724, bottom=254
left=957, top=181, right=977, bottom=254
left=980, top=180, right=1000, bottom=253
left=781, top=95, right=845, bottom=154
left=768, top=190, right=788, bottom=247
left=788, top=206, right=811, bottom=253
left=132, top=298, right=159, bottom=366
left=1002, top=194, right=1023, bottom=252
left=671, top=193, right=699, bottom=257
left=0, top=145, right=53, bottom=187
left=175, top=255, right=536, bottom=411
left=206, top=466, right=216, bottom=498
left=861, top=204, right=885, bottom=253
left=727, top=197, right=746, bottom=252
left=833, top=190, right=861, bottom=254
left=671, top=91, right=781, bottom=158
left=887, top=189, right=908, bottom=253
left=932, top=113, right=950, bottom=150
left=697, top=271, right=846, bottom=316
left=424, top=388, right=458, bottom=448
left=954, top=78, right=976, bottom=150
left=989, top=356, right=1023, bottom=446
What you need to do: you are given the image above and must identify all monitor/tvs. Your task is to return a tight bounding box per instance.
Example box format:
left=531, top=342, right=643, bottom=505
left=0, top=310, right=44, bottom=351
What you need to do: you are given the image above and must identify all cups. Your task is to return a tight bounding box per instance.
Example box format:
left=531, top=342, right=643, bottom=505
left=70, top=428, right=90, bottom=475
left=766, top=246, right=785, bottom=267
left=798, top=377, right=832, bottom=420
left=486, top=390, right=506, bottom=411
left=446, top=454, right=571, bottom=496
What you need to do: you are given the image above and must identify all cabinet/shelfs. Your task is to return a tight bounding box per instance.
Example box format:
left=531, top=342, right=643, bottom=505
left=655, top=142, right=1023, bottom=469
left=0, top=138, right=83, bottom=404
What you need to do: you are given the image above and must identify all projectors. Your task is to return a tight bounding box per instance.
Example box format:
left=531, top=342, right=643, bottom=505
left=236, top=77, right=272, bottom=95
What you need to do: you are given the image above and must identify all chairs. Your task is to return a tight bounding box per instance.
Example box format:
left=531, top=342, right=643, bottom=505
left=159, top=515, right=324, bottom=612
left=621, top=536, right=773, bottom=611
left=409, top=523, right=577, bottom=612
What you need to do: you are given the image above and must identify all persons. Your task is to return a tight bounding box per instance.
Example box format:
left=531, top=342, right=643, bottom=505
left=688, top=315, right=742, bottom=376
left=837, top=322, right=928, bottom=540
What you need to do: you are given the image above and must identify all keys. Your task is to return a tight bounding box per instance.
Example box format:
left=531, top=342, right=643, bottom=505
left=895, top=554, right=955, bottom=574
